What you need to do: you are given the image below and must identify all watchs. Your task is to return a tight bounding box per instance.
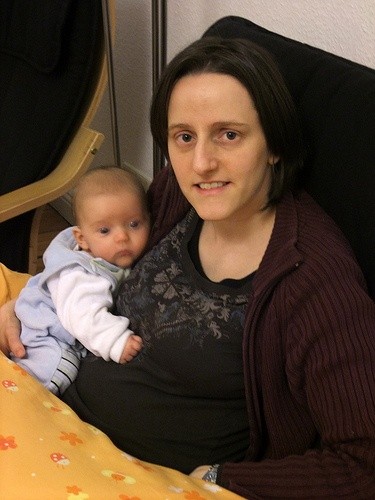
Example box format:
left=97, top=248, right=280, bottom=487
left=203, top=464, right=216, bottom=482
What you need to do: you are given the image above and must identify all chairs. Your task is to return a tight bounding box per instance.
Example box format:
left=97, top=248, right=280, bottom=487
left=0, top=0, right=115, bottom=274
left=146, top=15, right=375, bottom=302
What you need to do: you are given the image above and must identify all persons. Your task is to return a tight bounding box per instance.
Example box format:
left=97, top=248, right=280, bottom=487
left=2, top=37, right=375, bottom=499
left=11, top=165, right=149, bottom=395
